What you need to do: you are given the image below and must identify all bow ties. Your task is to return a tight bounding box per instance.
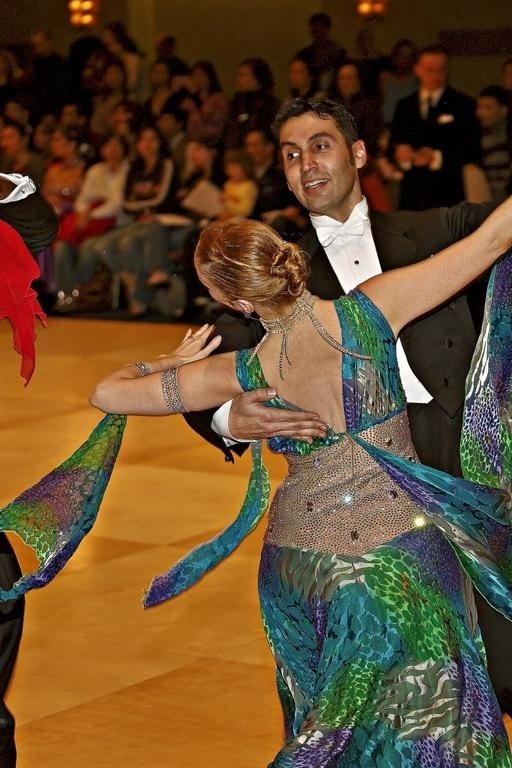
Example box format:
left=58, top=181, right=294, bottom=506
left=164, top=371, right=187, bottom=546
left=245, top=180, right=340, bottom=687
left=316, top=215, right=365, bottom=247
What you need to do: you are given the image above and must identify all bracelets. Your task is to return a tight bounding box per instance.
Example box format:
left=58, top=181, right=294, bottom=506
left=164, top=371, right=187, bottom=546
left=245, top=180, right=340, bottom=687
left=123, top=361, right=152, bottom=377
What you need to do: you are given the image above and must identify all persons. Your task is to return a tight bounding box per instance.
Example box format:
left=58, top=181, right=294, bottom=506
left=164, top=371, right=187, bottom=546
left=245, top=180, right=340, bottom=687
left=89, top=195, right=511, bottom=768
left=178, top=93, right=512, bottom=716
left=0, top=173, right=60, bottom=768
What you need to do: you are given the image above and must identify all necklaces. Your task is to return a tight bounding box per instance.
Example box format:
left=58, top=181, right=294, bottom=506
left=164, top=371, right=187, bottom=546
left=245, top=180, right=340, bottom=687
left=245, top=288, right=374, bottom=380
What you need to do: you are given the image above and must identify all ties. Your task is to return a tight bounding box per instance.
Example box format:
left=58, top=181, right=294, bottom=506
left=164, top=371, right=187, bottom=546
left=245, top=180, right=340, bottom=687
left=425, top=96, right=433, bottom=112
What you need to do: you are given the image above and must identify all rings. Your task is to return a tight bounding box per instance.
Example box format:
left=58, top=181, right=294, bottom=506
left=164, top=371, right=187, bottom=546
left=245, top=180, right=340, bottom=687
left=187, top=336, right=195, bottom=341
